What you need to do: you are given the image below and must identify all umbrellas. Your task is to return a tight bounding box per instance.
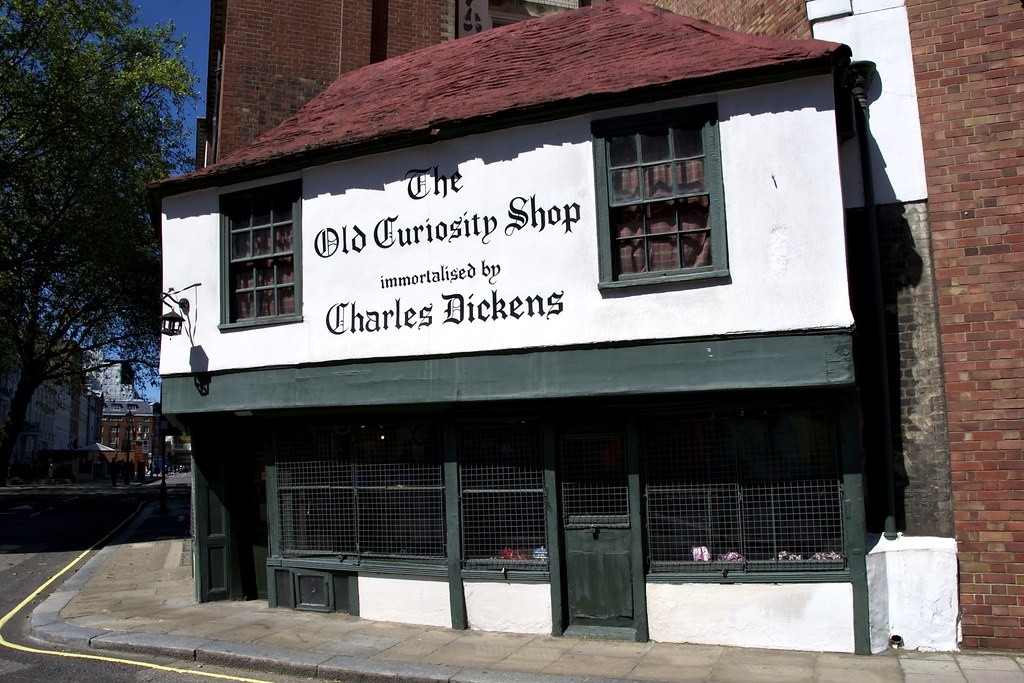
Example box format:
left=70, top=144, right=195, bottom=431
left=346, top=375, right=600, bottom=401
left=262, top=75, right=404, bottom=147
left=75, top=443, right=122, bottom=480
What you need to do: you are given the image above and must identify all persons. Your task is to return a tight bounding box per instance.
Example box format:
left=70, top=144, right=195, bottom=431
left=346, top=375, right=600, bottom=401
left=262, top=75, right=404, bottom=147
left=169, top=464, right=188, bottom=473
left=107, top=458, right=118, bottom=487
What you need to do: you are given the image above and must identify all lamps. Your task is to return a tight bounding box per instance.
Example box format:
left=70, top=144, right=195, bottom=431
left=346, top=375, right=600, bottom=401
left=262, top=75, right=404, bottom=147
left=157, top=283, right=202, bottom=336
